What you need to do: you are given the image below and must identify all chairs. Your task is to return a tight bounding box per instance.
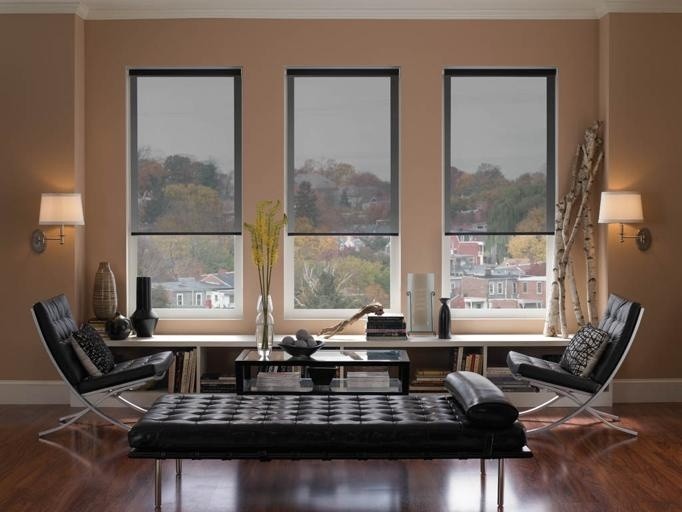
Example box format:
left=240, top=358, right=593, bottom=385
left=30, top=292, right=175, bottom=437
left=506, top=292, right=645, bottom=436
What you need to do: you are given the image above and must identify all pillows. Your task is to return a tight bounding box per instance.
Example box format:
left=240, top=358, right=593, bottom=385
left=558, top=322, right=611, bottom=378
left=70, top=320, right=115, bottom=377
left=444, top=371, right=519, bottom=425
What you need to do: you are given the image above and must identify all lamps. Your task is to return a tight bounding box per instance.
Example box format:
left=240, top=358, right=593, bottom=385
left=31, top=192, right=86, bottom=253
left=598, top=190, right=652, bottom=251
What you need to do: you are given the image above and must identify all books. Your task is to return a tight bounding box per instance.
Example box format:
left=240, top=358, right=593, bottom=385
left=461, top=353, right=482, bottom=376
left=256, top=381, right=300, bottom=386
left=255, top=366, right=307, bottom=380
left=167, top=349, right=197, bottom=394
left=201, top=372, right=236, bottom=394
left=409, top=364, right=452, bottom=394
left=365, top=311, right=407, bottom=339
left=488, top=367, right=538, bottom=393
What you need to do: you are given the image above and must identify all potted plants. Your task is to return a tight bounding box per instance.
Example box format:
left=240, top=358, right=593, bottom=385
left=243, top=200, right=289, bottom=357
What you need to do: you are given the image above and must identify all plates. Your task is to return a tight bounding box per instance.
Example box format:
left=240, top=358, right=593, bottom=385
left=278, top=340, right=326, bottom=359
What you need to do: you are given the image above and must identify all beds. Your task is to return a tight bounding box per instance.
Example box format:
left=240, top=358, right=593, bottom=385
left=126, top=395, right=534, bottom=512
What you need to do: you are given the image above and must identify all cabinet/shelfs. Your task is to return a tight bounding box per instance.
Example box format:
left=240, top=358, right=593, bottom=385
left=70, top=333, right=613, bottom=408
